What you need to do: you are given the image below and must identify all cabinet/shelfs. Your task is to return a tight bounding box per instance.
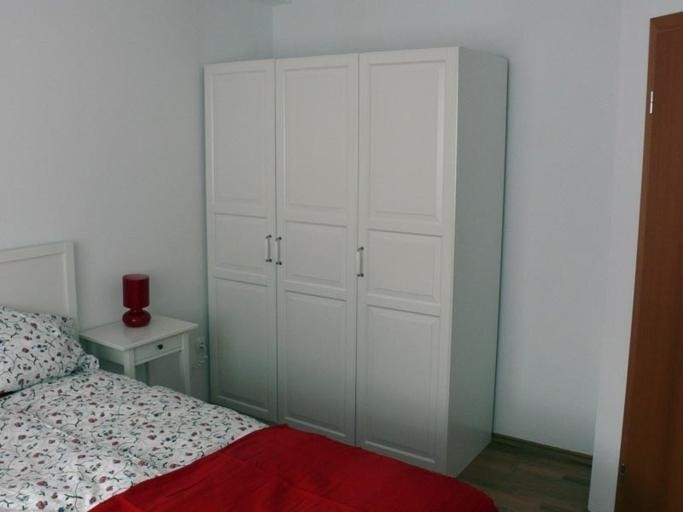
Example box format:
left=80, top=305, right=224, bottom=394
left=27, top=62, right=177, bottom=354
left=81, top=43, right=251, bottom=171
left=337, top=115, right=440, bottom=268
left=203, top=54, right=359, bottom=448
left=354, top=45, right=509, bottom=478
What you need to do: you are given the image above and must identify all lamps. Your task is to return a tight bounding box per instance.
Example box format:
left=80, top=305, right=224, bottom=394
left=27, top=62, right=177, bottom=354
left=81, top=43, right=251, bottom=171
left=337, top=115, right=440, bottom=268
left=122, top=274, right=151, bottom=326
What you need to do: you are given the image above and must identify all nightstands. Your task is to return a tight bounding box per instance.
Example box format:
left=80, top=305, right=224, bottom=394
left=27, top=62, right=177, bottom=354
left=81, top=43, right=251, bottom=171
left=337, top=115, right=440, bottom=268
left=79, top=314, right=198, bottom=395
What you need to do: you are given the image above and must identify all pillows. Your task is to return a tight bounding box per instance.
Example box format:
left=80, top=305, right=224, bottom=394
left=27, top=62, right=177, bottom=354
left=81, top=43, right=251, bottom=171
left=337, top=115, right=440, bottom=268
left=0, top=307, right=100, bottom=396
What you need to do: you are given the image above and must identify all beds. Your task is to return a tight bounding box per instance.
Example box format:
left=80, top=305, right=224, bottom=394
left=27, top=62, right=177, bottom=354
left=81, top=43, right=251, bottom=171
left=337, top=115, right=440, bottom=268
left=0, top=240, right=502, bottom=511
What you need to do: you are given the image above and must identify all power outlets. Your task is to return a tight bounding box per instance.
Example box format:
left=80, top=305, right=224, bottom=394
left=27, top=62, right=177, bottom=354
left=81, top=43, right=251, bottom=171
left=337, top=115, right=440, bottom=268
left=196, top=337, right=206, bottom=352
